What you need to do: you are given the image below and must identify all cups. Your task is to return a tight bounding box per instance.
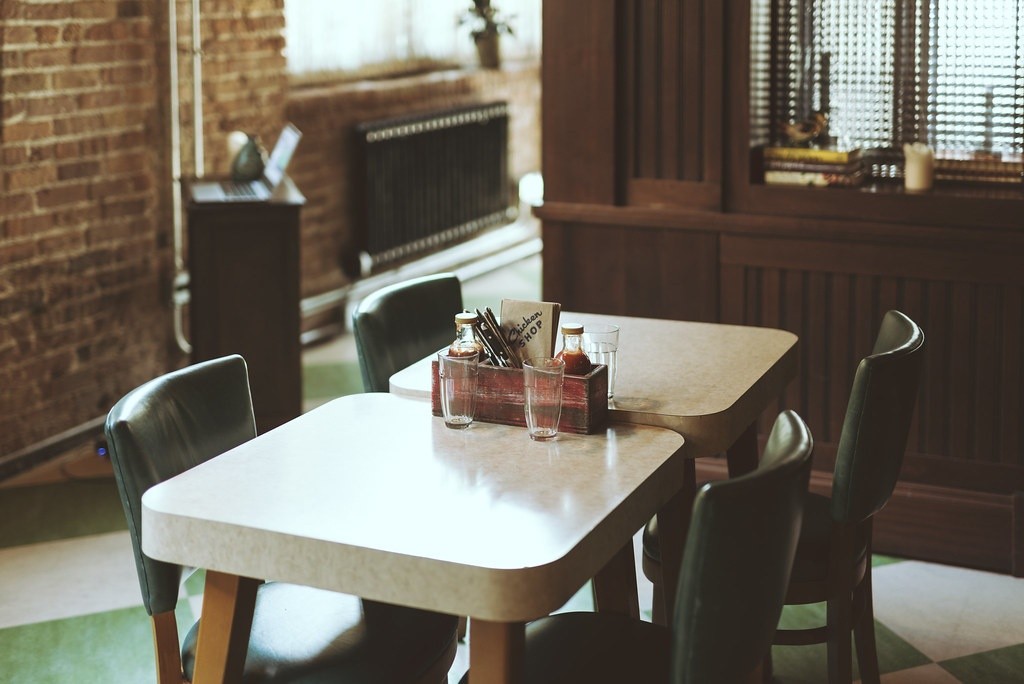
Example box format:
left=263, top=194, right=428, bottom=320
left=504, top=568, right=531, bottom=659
left=522, top=357, right=565, bottom=443
left=438, top=346, right=479, bottom=429
left=583, top=323, right=620, bottom=401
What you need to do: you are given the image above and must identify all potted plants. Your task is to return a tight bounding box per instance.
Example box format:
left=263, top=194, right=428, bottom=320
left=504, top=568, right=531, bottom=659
left=457, top=6, right=520, bottom=71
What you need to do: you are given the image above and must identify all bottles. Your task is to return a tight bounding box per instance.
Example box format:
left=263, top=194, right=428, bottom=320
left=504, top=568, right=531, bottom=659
left=555, top=323, right=591, bottom=376
left=449, top=313, right=485, bottom=363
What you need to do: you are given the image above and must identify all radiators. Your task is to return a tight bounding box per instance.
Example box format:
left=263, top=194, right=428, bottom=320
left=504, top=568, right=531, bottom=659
left=354, top=100, right=516, bottom=272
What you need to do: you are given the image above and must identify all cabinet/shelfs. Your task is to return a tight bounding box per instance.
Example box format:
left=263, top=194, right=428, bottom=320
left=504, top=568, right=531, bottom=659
left=542, top=136, right=1024, bottom=577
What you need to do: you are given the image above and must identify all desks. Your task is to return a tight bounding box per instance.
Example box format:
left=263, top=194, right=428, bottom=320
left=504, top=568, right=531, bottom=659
left=178, top=167, right=309, bottom=442
left=140, top=387, right=686, bottom=684
left=388, top=310, right=800, bottom=682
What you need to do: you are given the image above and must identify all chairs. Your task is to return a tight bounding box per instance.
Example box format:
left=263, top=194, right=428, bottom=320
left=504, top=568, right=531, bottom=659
left=642, top=309, right=926, bottom=684
left=456, top=406, right=815, bottom=684
left=103, top=353, right=460, bottom=684
left=351, top=273, right=469, bottom=395
